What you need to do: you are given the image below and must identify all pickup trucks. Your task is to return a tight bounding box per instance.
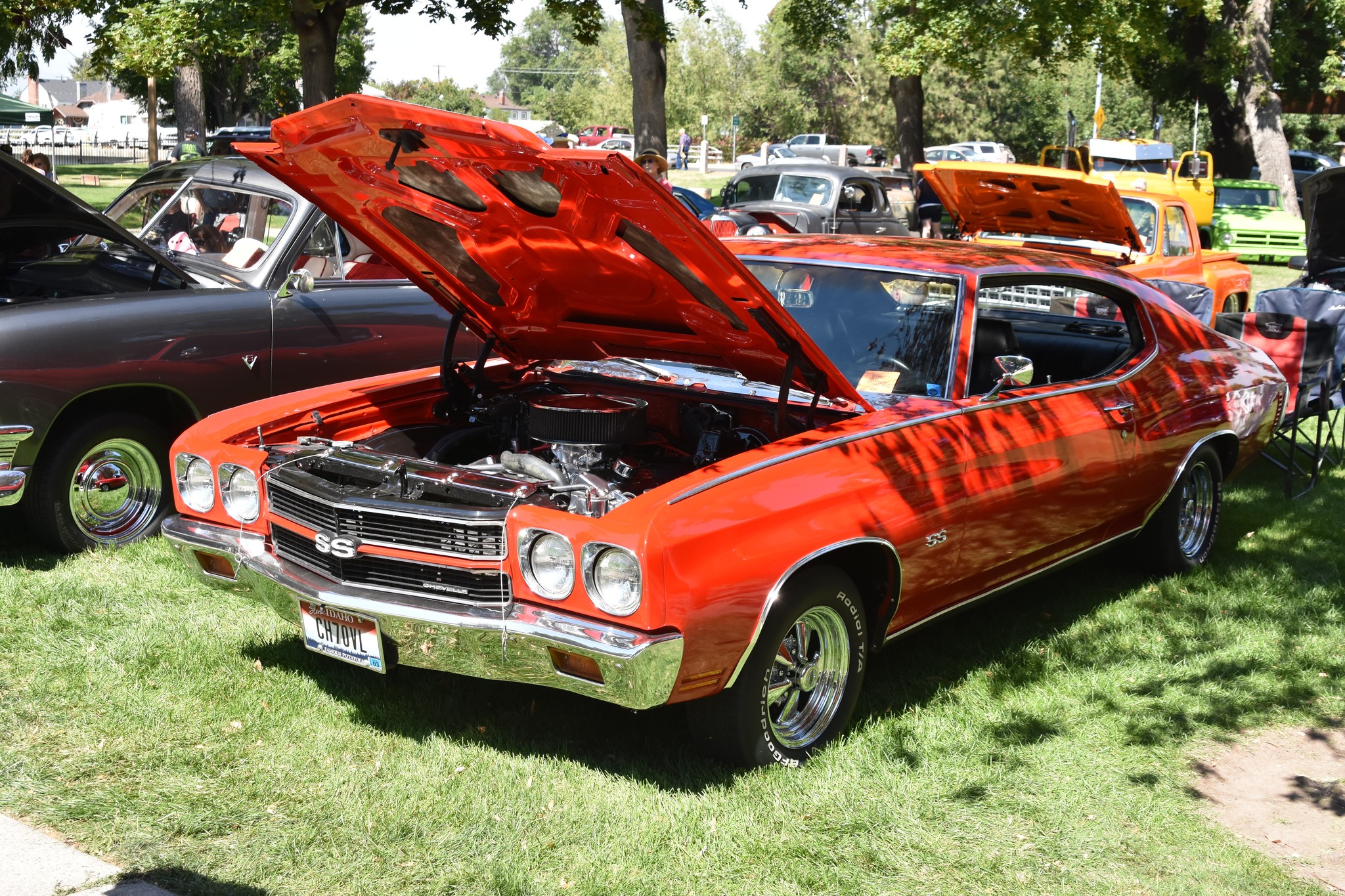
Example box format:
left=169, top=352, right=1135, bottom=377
left=910, top=159, right=1254, bottom=339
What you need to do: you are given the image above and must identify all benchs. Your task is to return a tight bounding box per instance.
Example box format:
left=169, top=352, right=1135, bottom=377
left=857, top=317, right=1129, bottom=385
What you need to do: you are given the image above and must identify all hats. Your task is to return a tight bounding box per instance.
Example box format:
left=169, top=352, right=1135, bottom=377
left=535, top=134, right=554, bottom=146
left=875, top=154, right=886, bottom=161
left=633, top=148, right=668, bottom=173
left=184, top=127, right=199, bottom=135
left=208, top=139, right=229, bottom=150
left=678, top=128, right=685, bottom=133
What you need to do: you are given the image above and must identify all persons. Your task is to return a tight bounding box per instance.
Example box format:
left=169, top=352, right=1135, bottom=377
left=866, top=154, right=886, bottom=167
left=677, top=128, right=691, bottom=170
left=848, top=159, right=858, bottom=168
left=22, top=148, right=59, bottom=185
left=916, top=177, right=943, bottom=240
left=156, top=127, right=246, bottom=254
left=633, top=148, right=672, bottom=193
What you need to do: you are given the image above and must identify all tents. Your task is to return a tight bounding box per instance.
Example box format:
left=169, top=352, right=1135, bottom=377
left=0, top=92, right=56, bottom=183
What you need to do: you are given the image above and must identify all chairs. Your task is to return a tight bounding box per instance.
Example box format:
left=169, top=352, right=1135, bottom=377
left=247, top=249, right=406, bottom=280
left=1142, top=280, right=1345, bottom=500
left=925, top=313, right=1024, bottom=399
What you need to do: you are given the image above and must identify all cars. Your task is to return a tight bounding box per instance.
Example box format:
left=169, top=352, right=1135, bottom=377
left=576, top=124, right=1343, bottom=270
left=0, top=147, right=504, bottom=558
left=157, top=93, right=1289, bottom=771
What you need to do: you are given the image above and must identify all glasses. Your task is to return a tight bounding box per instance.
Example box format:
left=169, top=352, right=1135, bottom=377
left=192, top=238, right=206, bottom=246
left=210, top=149, right=220, bottom=154
left=640, top=158, right=657, bottom=165
